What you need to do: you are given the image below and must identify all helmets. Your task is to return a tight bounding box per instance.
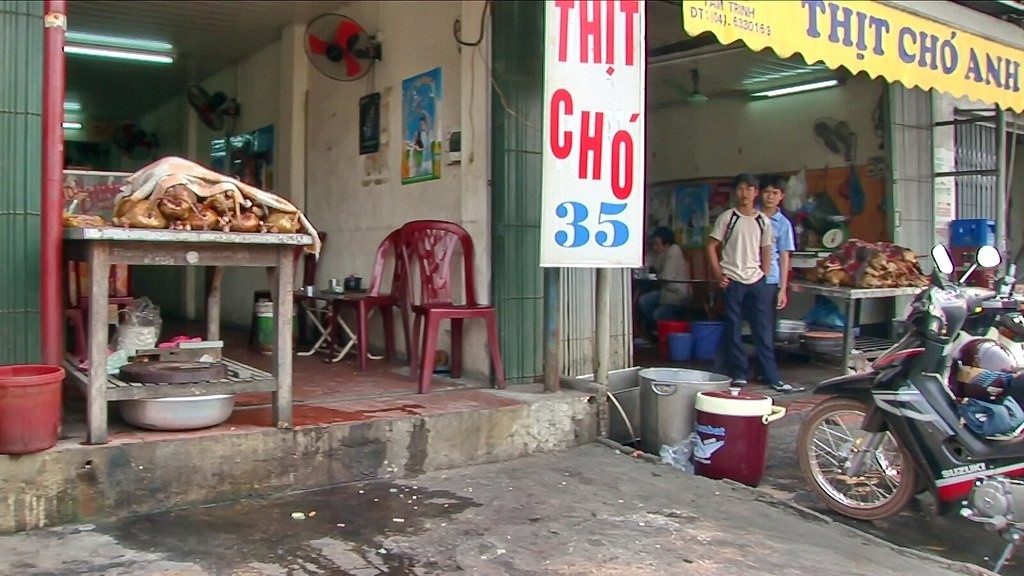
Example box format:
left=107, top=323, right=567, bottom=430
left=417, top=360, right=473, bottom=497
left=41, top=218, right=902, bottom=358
left=948, top=338, right=1019, bottom=403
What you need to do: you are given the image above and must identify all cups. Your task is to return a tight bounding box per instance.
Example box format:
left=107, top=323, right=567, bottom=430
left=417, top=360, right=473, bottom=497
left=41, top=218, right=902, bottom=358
left=306, top=285, right=313, bottom=297
left=344, top=277, right=362, bottom=292
left=329, top=278, right=336, bottom=289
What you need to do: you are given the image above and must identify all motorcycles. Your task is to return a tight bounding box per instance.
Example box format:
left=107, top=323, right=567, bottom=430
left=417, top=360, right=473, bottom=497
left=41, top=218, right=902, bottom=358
left=796, top=244, right=1023, bottom=576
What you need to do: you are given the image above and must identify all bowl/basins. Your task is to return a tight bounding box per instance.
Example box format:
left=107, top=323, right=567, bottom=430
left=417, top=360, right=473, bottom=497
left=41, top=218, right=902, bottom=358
left=774, top=319, right=809, bottom=342
left=119, top=394, right=234, bottom=430
left=805, top=336, right=843, bottom=353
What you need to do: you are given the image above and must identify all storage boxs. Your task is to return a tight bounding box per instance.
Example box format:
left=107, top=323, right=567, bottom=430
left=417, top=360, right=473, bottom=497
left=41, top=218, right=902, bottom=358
left=950, top=217, right=995, bottom=288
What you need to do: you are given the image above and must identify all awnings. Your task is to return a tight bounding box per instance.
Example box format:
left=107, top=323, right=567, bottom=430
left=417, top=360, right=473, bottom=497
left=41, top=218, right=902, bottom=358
left=672, top=0, right=1024, bottom=114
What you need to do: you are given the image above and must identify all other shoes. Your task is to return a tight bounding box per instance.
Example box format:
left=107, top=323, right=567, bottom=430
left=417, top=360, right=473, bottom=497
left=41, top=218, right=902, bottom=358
left=731, top=379, right=747, bottom=387
left=634, top=338, right=652, bottom=344
left=770, top=380, right=792, bottom=391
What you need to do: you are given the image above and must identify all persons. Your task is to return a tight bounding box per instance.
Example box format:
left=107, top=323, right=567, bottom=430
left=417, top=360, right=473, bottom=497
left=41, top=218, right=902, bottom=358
left=411, top=111, right=430, bottom=174
left=632, top=227, right=694, bottom=349
left=705, top=172, right=796, bottom=391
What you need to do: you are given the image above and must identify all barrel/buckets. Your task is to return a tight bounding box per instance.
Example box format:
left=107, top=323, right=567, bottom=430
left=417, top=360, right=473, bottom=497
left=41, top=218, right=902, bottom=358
left=116, top=325, right=156, bottom=356
left=692, top=321, right=724, bottom=361
left=657, top=321, right=690, bottom=357
left=256, top=302, right=297, bottom=355
left=639, top=367, right=732, bottom=458
left=667, top=333, right=691, bottom=361
left=0, top=365, right=66, bottom=454
left=692, top=387, right=786, bottom=488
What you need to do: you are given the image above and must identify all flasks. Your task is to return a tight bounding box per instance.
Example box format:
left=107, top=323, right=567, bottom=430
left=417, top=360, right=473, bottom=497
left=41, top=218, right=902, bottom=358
left=431, top=140, right=441, bottom=178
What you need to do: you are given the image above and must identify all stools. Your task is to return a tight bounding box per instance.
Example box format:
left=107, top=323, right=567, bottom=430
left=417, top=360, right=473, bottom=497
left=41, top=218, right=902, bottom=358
left=65, top=306, right=86, bottom=360
left=79, top=296, right=133, bottom=335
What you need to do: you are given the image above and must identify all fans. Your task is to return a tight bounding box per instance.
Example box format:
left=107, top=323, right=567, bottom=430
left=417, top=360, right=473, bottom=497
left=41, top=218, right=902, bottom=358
left=810, top=116, right=857, bottom=159
left=304, top=12, right=383, bottom=82
left=658, top=70, right=767, bottom=109
left=184, top=84, right=239, bottom=131
left=111, top=122, right=161, bottom=160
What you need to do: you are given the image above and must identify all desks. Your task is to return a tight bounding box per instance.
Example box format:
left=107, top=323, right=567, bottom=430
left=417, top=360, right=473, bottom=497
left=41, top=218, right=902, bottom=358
left=292, top=289, right=383, bottom=362
left=743, top=281, right=935, bottom=374
left=63, top=225, right=315, bottom=445
left=658, top=277, right=724, bottom=319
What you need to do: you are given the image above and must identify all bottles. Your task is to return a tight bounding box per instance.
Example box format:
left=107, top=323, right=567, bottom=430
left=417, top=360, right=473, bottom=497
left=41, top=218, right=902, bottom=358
left=998, top=263, right=1016, bottom=299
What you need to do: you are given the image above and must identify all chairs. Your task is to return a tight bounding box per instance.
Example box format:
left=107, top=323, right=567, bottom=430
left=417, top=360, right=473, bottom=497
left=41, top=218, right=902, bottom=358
left=327, top=228, right=415, bottom=369
left=251, top=231, right=326, bottom=348
left=401, top=219, right=505, bottom=394
